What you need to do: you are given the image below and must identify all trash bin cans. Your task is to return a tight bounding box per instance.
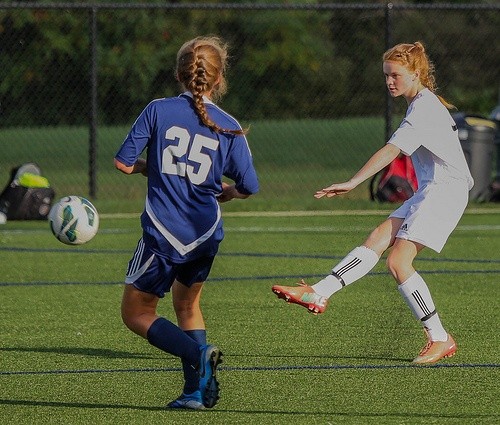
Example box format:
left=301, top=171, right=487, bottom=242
left=449, top=113, right=500, bottom=204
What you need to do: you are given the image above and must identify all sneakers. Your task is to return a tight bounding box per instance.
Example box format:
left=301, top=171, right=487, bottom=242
left=190, top=343, right=225, bottom=408
left=271, top=279, right=327, bottom=315
left=167, top=388, right=204, bottom=410
left=410, top=327, right=456, bottom=364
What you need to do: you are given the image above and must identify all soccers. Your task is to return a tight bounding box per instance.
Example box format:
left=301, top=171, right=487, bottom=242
left=48, top=196, right=99, bottom=245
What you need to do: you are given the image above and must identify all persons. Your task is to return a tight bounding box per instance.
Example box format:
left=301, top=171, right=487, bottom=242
left=272, top=40, right=473, bottom=363
left=114, top=38, right=259, bottom=411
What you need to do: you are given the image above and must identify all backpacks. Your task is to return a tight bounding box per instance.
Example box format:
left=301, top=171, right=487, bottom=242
left=0, top=162, right=56, bottom=222
left=370, top=157, right=419, bottom=202
